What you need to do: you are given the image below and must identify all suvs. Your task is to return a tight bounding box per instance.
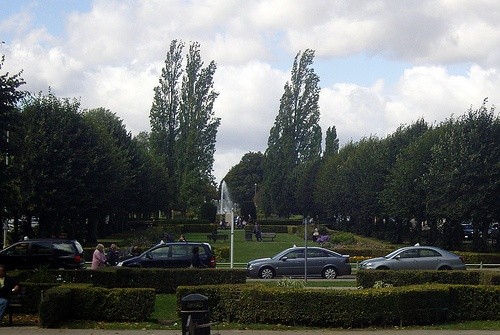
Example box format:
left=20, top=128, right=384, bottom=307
left=121, top=241, right=216, bottom=269
left=0, top=238, right=87, bottom=270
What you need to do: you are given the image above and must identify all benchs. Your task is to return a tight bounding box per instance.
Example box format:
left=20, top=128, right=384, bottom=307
left=4, top=284, right=29, bottom=325
left=262, top=233, right=276, bottom=242
left=207, top=235, right=228, bottom=242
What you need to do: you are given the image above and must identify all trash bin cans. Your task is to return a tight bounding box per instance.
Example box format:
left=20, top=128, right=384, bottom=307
left=245, top=229, right=253, bottom=241
left=182, top=294, right=210, bottom=334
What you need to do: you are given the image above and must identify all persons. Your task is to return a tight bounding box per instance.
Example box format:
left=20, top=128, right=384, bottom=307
left=191, top=246, right=206, bottom=269
left=180, top=235, right=187, bottom=243
left=92, top=244, right=109, bottom=270
left=0, top=265, right=19, bottom=320
left=107, top=244, right=119, bottom=266
left=212, top=214, right=263, bottom=242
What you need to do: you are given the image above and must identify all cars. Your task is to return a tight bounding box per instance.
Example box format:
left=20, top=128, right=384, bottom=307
left=246, top=244, right=351, bottom=280
left=461, top=224, right=473, bottom=239
left=359, top=243, right=466, bottom=271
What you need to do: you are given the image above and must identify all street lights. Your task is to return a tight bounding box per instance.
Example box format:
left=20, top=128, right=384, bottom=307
left=254, top=182, right=257, bottom=195
